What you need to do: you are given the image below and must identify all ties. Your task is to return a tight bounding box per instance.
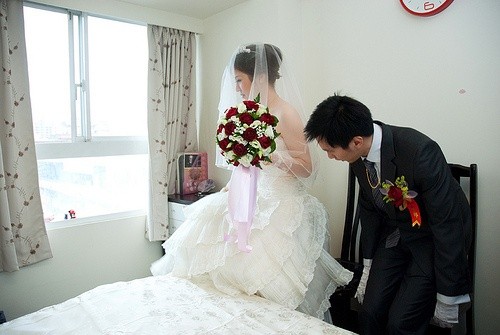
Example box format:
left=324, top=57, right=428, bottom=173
left=363, top=159, right=401, bottom=249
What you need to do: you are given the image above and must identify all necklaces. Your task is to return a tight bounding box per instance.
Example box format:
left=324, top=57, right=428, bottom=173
left=366, top=169, right=380, bottom=189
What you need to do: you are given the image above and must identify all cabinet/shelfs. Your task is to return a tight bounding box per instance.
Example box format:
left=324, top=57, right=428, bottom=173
left=162, top=192, right=215, bottom=256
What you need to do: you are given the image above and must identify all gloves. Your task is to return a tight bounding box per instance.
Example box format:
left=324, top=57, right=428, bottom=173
left=433, top=300, right=459, bottom=329
left=354, top=266, right=371, bottom=304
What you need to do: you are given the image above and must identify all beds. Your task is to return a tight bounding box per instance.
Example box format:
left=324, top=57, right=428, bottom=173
left=0, top=272, right=357, bottom=335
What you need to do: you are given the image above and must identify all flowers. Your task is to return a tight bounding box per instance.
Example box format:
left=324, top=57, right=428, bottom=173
left=378, top=176, right=418, bottom=211
left=216, top=92, right=282, bottom=170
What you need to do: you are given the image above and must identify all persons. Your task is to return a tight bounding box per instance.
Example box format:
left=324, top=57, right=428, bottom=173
left=150, top=42, right=354, bottom=325
left=304, top=96, right=473, bottom=335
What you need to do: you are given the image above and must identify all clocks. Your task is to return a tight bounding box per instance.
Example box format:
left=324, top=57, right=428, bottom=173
left=399, top=0, right=454, bottom=17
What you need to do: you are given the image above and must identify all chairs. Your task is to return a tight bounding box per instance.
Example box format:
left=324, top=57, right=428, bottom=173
left=330, top=157, right=478, bottom=335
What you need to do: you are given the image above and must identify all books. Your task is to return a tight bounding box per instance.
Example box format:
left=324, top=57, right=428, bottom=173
left=175, top=150, right=209, bottom=196
